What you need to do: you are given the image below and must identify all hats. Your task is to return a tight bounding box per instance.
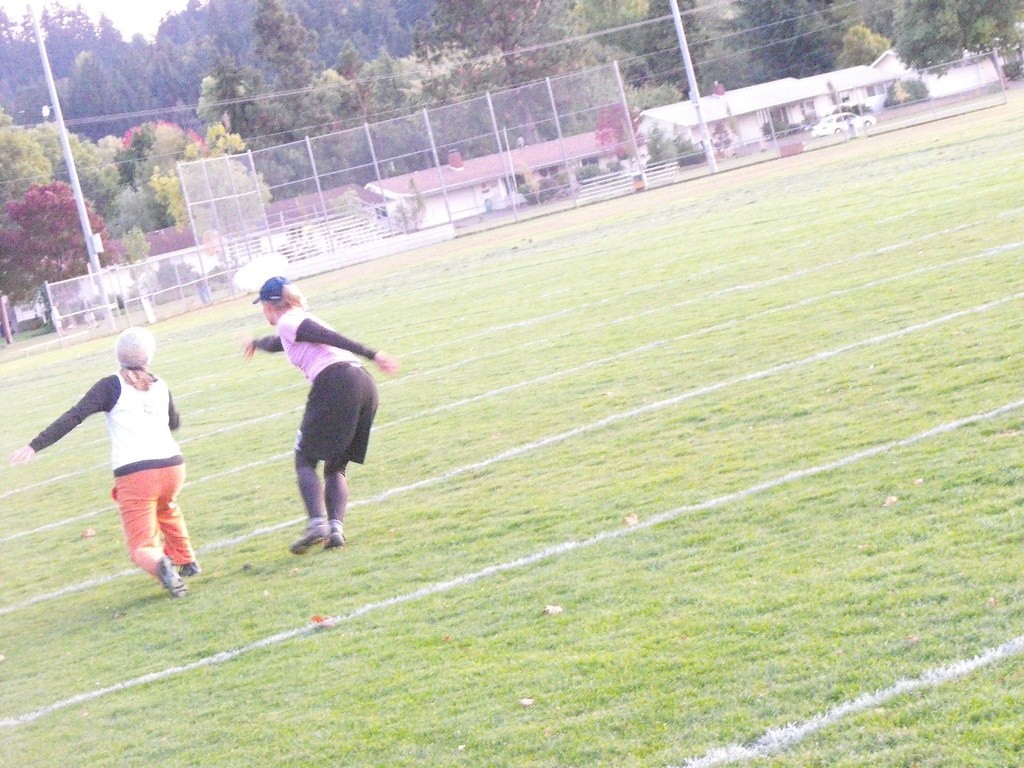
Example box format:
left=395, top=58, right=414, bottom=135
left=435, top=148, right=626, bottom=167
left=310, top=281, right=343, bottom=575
left=252, top=276, right=292, bottom=304
left=115, top=326, right=155, bottom=367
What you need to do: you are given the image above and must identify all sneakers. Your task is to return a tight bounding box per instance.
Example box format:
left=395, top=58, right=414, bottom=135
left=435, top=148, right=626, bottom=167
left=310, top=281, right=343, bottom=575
left=289, top=522, right=327, bottom=554
left=323, top=528, right=346, bottom=549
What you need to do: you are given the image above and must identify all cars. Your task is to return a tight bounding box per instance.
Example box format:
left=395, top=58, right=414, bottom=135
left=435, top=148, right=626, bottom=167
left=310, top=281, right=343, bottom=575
left=811, top=112, right=876, bottom=137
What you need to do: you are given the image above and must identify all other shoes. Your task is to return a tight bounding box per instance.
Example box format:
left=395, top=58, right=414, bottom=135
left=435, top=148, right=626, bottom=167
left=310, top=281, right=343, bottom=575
left=156, top=555, right=187, bottom=597
left=177, top=560, right=201, bottom=577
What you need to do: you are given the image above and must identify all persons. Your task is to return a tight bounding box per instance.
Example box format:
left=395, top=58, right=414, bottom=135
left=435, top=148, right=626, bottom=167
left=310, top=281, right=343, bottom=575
left=8, top=326, right=202, bottom=599
left=243, top=277, right=397, bottom=557
